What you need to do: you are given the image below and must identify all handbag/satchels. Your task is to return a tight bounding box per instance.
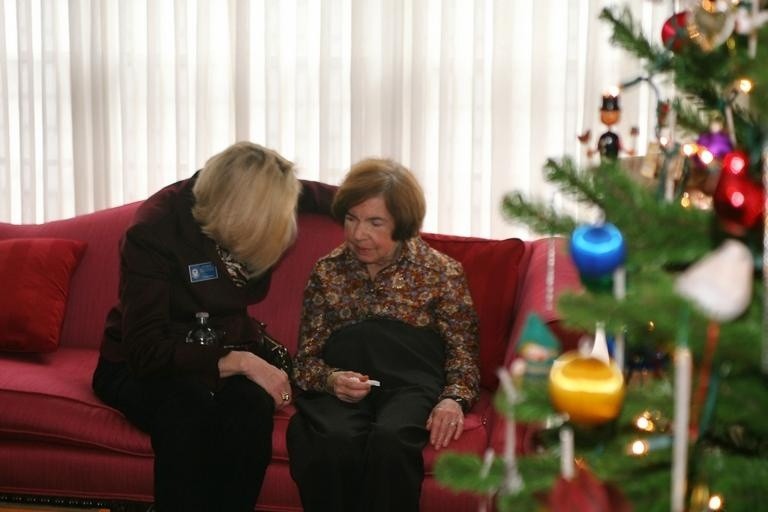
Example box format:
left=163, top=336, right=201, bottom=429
left=187, top=314, right=292, bottom=399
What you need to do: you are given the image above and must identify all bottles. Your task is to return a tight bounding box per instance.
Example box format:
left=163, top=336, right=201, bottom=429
left=185, top=312, right=218, bottom=345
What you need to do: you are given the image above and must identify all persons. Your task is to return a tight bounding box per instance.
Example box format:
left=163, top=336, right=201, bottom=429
left=281, top=156, right=485, bottom=510
left=91, top=139, right=343, bottom=512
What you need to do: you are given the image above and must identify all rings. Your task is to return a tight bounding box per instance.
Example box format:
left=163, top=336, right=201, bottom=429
left=448, top=422, right=458, bottom=426
left=282, top=392, right=291, bottom=402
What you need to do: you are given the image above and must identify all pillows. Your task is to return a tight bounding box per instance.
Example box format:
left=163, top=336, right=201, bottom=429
left=418, top=231, right=525, bottom=394
left=0, top=238, right=88, bottom=356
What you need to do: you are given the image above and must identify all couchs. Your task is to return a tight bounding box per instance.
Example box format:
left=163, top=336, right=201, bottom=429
left=0, top=197, right=589, bottom=512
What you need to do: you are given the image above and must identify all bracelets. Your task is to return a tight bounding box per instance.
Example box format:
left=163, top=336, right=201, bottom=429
left=447, top=396, right=469, bottom=413
